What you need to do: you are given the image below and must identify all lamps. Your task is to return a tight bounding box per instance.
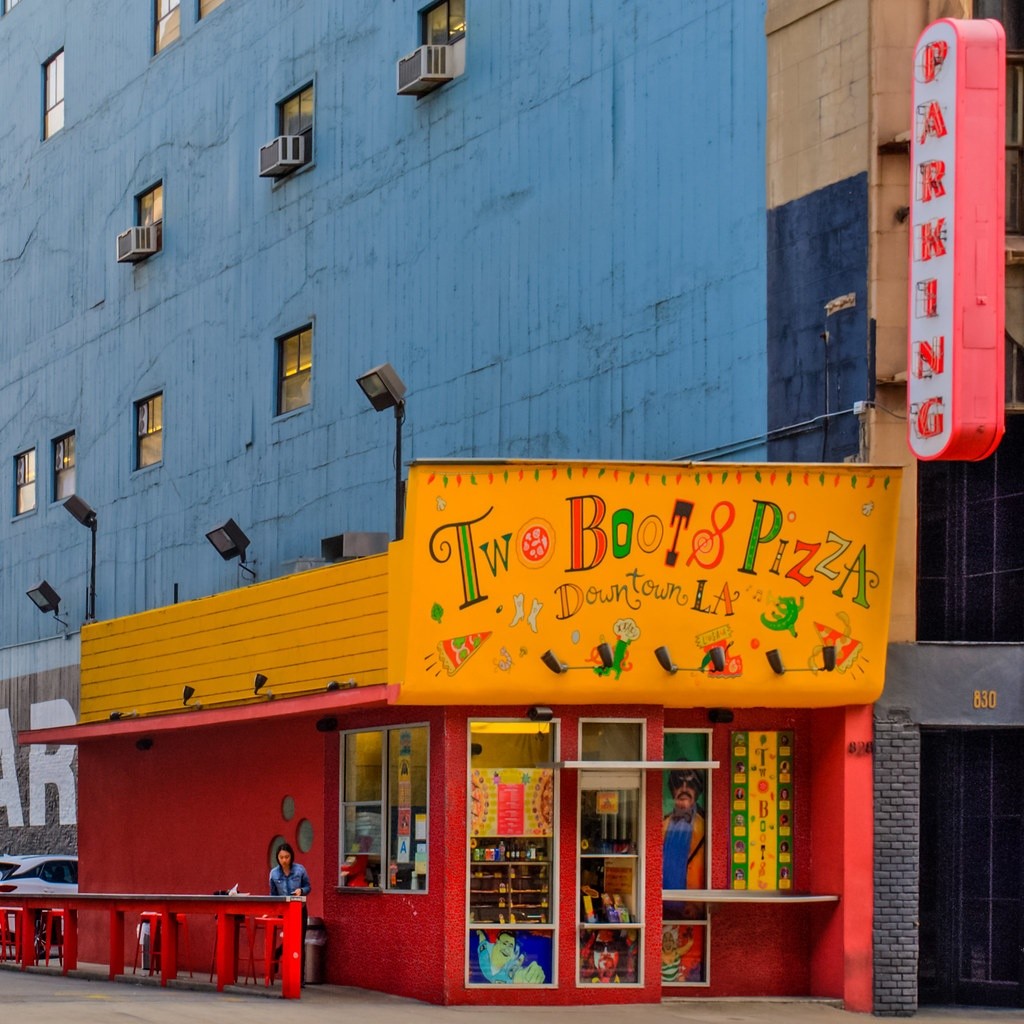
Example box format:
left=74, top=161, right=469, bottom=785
left=326, top=678, right=355, bottom=691
left=355, top=361, right=408, bottom=539
left=182, top=685, right=201, bottom=709
left=539, top=649, right=569, bottom=673
left=205, top=518, right=257, bottom=584
left=254, top=673, right=273, bottom=700
left=592, top=642, right=613, bottom=676
left=654, top=646, right=679, bottom=674
left=765, top=648, right=785, bottom=675
left=709, top=646, right=725, bottom=673
left=26, top=580, right=68, bottom=633
left=110, top=710, right=138, bottom=721
left=819, top=646, right=836, bottom=672
left=61, top=495, right=98, bottom=622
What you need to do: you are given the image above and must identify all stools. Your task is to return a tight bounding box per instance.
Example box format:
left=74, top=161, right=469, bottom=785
left=245, top=918, right=283, bottom=988
left=0, top=907, right=23, bottom=964
left=35, top=909, right=64, bottom=967
left=210, top=913, right=257, bottom=985
left=132, top=911, right=194, bottom=978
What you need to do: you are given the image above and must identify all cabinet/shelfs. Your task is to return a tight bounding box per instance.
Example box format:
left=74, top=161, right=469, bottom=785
left=470, top=862, right=552, bottom=924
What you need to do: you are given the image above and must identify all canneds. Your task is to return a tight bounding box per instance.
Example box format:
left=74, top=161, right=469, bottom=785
left=473, top=848, right=500, bottom=861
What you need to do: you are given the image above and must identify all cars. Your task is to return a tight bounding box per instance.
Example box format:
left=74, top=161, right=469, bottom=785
left=0, top=852, right=78, bottom=944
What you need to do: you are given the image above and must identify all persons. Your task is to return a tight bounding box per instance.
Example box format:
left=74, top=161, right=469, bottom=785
left=54, top=866, right=69, bottom=882
left=268, top=841, right=311, bottom=989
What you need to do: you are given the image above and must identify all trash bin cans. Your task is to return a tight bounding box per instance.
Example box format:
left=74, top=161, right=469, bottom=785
left=304, top=916, right=327, bottom=983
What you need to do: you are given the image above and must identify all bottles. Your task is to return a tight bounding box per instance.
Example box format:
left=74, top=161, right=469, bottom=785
left=499, top=840, right=543, bottom=862
left=411, top=871, right=419, bottom=889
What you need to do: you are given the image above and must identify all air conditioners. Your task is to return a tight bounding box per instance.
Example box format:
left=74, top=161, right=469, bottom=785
left=397, top=45, right=454, bottom=94
left=116, top=226, right=158, bottom=261
left=259, top=136, right=304, bottom=175
